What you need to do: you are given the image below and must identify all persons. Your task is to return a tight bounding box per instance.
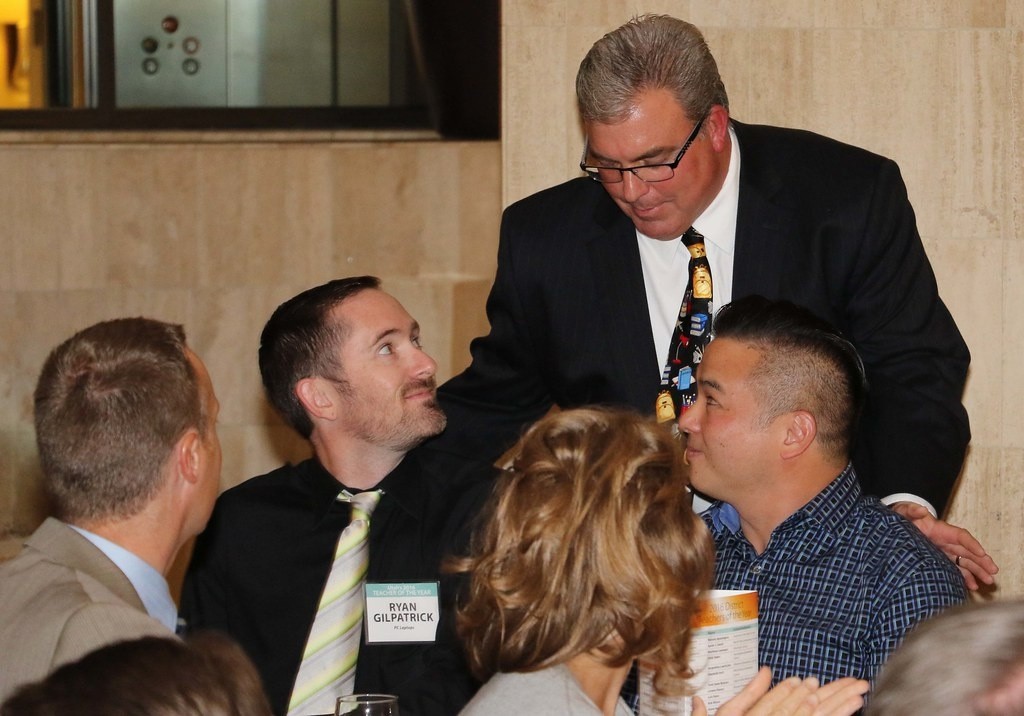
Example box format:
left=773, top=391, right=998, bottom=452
left=174, top=276, right=498, bottom=716
left=0, top=316, right=223, bottom=705
left=440, top=301, right=1024, bottom=716
left=429, top=13, right=999, bottom=594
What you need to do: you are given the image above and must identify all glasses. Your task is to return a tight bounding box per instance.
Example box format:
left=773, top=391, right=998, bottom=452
left=580, top=112, right=709, bottom=183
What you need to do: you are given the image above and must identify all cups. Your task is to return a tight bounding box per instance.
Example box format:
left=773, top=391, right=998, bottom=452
left=334, top=693, right=399, bottom=716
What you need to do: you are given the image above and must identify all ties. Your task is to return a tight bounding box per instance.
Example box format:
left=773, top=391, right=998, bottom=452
left=287, top=489, right=385, bottom=716
left=657, top=226, right=713, bottom=441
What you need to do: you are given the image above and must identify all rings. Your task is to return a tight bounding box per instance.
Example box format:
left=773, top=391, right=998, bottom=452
left=956, top=555, right=962, bottom=566
